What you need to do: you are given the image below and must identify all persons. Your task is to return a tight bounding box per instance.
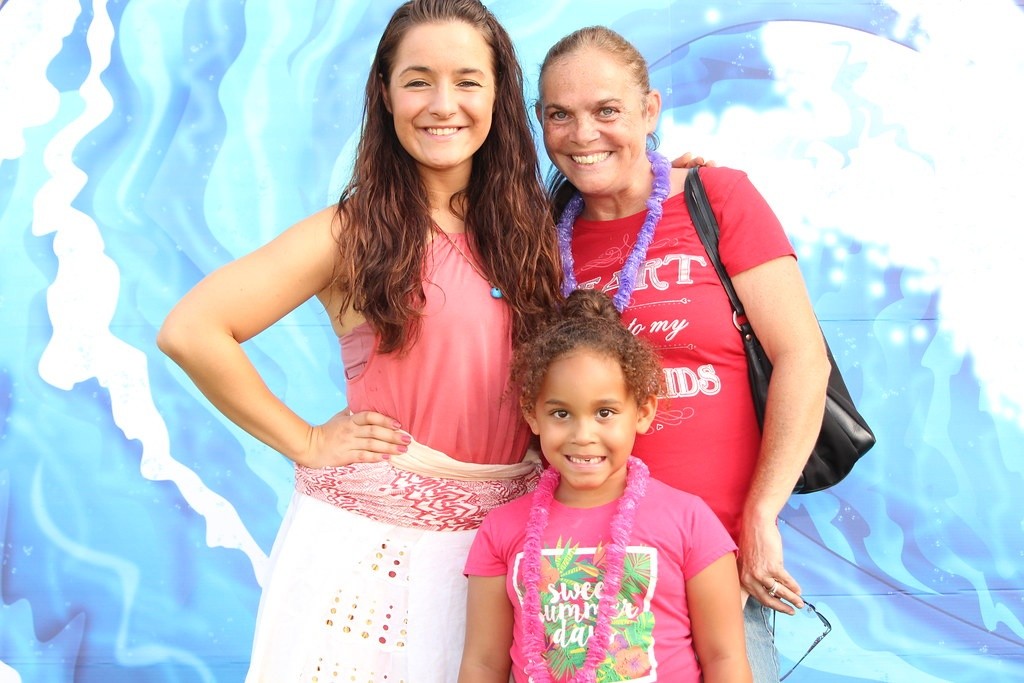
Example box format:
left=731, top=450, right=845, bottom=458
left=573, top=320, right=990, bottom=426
left=534, top=25, right=831, bottom=683
left=156, top=0, right=560, bottom=683
left=456, top=288, right=755, bottom=683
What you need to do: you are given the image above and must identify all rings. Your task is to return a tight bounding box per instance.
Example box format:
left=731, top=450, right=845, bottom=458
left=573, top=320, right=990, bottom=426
left=766, top=581, right=779, bottom=597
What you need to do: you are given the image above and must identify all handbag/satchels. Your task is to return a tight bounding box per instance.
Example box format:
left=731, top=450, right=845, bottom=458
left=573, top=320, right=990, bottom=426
left=684, top=165, right=875, bottom=494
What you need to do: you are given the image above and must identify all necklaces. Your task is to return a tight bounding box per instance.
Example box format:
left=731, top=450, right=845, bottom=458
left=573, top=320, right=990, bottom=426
left=428, top=213, right=503, bottom=298
left=557, top=150, right=671, bottom=313
left=521, top=455, right=650, bottom=683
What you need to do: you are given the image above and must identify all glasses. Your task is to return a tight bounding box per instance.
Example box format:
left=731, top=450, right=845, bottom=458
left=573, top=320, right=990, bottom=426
left=772, top=594, right=832, bottom=681
